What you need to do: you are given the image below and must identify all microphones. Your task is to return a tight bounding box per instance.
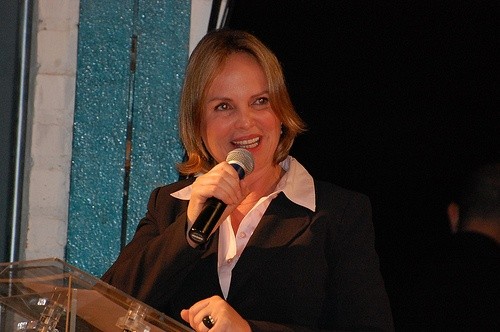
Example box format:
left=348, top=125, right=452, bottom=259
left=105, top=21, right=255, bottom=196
left=189, top=148, right=255, bottom=244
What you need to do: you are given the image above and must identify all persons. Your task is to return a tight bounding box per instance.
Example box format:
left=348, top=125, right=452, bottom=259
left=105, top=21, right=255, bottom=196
left=101, top=29, right=393, bottom=332
left=393, top=180, right=500, bottom=332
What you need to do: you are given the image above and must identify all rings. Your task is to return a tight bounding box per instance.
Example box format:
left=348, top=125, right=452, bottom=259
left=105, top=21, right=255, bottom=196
left=202, top=317, right=214, bottom=330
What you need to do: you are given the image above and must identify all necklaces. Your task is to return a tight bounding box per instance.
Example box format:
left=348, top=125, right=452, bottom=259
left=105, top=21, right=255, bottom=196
left=238, top=168, right=283, bottom=206
